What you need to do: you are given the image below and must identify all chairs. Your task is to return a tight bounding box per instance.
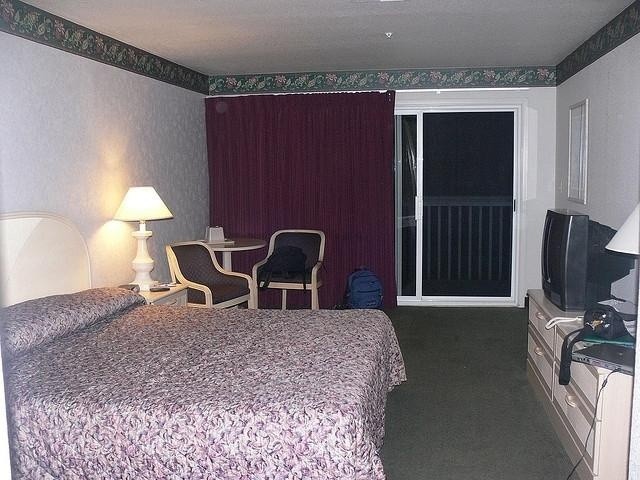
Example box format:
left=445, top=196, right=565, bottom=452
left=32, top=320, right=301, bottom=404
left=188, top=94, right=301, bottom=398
left=252, top=228, right=326, bottom=312
left=165, top=241, right=254, bottom=308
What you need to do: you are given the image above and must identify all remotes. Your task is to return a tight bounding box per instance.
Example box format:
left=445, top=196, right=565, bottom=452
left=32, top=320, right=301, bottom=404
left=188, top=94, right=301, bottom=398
left=150, top=288, right=170, bottom=292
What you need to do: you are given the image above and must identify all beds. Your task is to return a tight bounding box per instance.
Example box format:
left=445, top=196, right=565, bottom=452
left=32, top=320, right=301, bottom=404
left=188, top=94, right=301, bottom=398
left=0, top=212, right=406, bottom=480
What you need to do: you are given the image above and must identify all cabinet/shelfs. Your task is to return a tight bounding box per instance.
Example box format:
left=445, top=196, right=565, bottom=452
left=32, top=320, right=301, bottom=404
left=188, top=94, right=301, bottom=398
left=525, top=288, right=633, bottom=480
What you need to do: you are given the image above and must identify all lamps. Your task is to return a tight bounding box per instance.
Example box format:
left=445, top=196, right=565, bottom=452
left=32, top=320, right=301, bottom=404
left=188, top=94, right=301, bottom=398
left=112, top=184, right=173, bottom=292
left=604, top=202, right=638, bottom=326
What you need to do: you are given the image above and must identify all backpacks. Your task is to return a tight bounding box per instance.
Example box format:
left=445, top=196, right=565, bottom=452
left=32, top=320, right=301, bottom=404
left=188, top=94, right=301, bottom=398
left=341, top=270, right=384, bottom=310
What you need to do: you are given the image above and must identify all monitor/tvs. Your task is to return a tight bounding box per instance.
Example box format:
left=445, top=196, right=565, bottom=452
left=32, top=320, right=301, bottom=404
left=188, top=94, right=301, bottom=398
left=542, top=208, right=635, bottom=311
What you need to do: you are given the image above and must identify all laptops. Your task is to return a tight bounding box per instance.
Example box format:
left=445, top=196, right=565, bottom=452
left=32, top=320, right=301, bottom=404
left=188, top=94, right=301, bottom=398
left=571, top=342, right=635, bottom=377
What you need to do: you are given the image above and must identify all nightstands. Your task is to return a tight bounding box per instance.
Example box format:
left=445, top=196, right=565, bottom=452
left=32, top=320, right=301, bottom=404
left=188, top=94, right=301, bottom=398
left=143, top=285, right=190, bottom=309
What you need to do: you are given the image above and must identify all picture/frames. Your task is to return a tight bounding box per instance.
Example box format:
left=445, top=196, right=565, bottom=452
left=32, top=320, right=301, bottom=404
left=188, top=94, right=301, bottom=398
left=568, top=97, right=589, bottom=208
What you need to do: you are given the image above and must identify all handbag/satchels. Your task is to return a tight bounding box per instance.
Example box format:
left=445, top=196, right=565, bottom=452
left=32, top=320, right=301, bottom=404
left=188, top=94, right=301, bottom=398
left=264, top=246, right=305, bottom=279
left=582, top=305, right=625, bottom=339
